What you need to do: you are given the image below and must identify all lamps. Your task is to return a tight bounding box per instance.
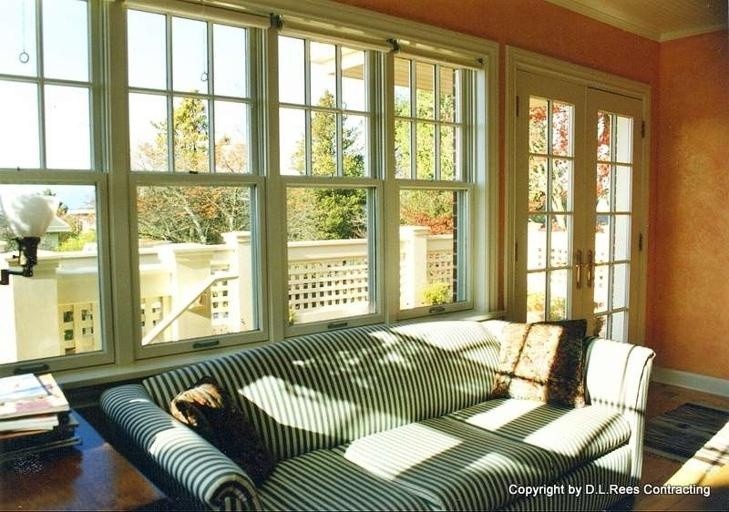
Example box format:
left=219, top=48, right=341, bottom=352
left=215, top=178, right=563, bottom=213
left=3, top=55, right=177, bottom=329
left=0, top=185, right=59, bottom=311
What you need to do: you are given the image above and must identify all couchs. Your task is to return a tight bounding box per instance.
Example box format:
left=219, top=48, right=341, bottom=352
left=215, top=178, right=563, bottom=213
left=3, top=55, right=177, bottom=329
left=97, top=316, right=655, bottom=512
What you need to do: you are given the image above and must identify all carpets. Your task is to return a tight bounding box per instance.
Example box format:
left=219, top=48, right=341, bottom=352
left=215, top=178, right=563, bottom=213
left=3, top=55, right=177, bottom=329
left=642, top=401, right=729, bottom=474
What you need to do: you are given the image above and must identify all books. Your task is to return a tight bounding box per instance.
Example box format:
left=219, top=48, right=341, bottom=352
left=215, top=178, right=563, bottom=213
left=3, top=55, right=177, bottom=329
left=0, top=370, right=85, bottom=465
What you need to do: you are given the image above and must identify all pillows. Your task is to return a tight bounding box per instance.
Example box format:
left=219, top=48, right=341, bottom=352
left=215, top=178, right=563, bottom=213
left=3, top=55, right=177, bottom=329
left=486, top=320, right=590, bottom=412
left=169, top=377, right=282, bottom=494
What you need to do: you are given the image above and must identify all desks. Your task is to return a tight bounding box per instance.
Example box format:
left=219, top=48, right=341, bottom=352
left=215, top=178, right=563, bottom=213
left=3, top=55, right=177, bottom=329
left=0, top=400, right=168, bottom=511
